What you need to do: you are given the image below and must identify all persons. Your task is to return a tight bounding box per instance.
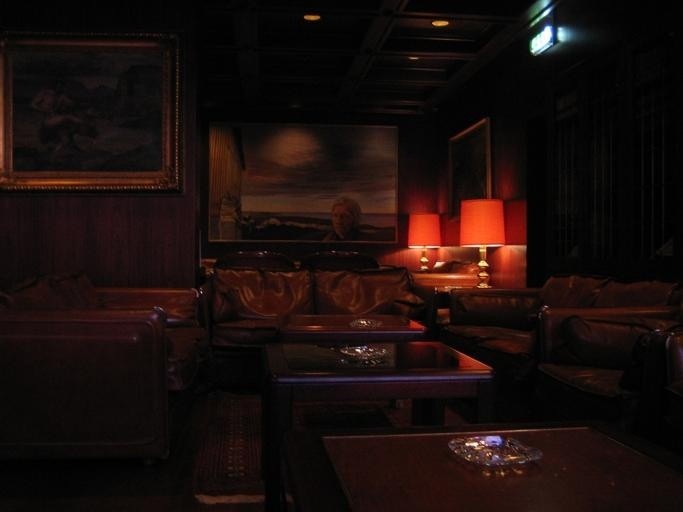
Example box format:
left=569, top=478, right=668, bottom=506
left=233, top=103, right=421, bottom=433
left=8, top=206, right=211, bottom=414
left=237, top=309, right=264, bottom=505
left=27, top=76, right=95, bottom=162
left=323, top=194, right=374, bottom=240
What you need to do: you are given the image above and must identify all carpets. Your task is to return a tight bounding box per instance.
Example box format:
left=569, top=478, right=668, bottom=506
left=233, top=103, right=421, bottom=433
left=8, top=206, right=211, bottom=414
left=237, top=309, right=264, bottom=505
left=193, top=383, right=467, bottom=505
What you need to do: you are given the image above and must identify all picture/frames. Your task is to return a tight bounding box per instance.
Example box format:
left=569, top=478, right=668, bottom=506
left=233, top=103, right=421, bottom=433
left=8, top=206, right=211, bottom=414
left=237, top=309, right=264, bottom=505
left=448, top=115, right=493, bottom=218
left=0, top=32, right=190, bottom=196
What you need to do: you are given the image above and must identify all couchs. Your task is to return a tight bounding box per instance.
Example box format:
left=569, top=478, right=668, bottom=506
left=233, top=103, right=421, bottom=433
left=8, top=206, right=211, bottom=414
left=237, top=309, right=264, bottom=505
left=537, top=304, right=683, bottom=428
left=193, top=247, right=430, bottom=344
left=1, top=273, right=201, bottom=495
left=445, top=274, right=677, bottom=424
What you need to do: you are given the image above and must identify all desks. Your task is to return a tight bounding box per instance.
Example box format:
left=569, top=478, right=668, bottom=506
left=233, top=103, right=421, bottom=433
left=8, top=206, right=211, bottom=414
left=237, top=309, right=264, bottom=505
left=214, top=313, right=428, bottom=347
left=412, top=273, right=491, bottom=319
left=258, top=337, right=493, bottom=438
left=321, top=425, right=683, bottom=511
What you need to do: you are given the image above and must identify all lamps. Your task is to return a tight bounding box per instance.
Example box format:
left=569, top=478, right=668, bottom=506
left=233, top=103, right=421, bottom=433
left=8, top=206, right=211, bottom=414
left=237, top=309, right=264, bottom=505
left=407, top=213, right=442, bottom=271
left=459, top=198, right=504, bottom=288
